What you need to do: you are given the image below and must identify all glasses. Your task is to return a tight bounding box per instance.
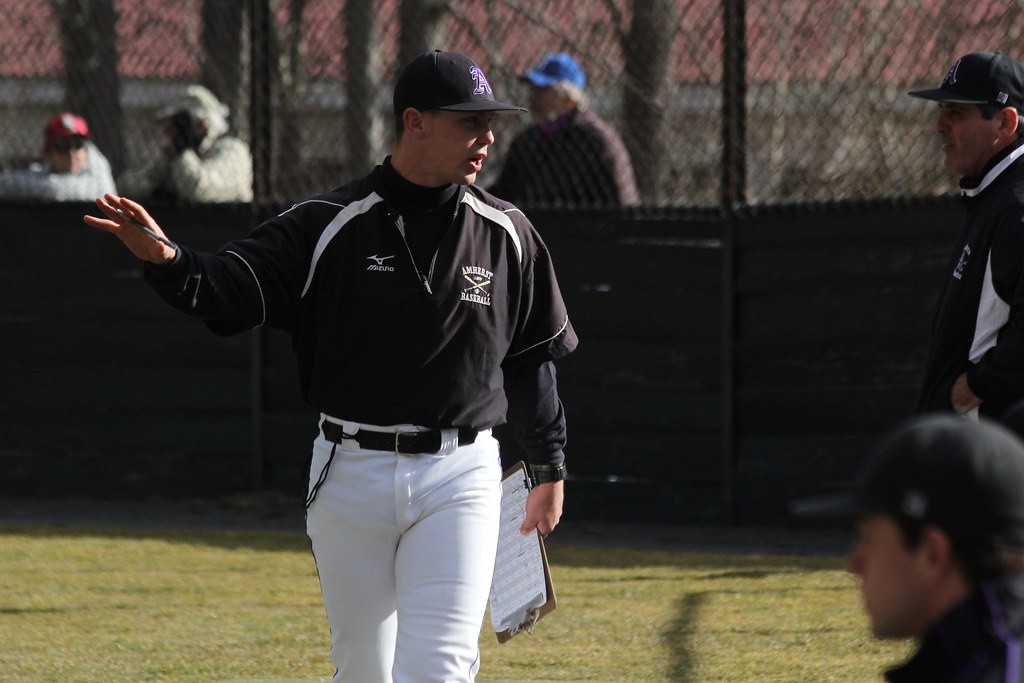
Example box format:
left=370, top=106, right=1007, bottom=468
left=48, top=135, right=84, bottom=151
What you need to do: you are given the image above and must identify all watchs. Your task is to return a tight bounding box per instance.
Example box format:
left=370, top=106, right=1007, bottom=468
left=526, top=463, right=569, bottom=489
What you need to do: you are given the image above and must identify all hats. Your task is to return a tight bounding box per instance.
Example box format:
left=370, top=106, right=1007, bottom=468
left=515, top=51, right=587, bottom=89
left=45, top=112, right=90, bottom=143
left=907, top=51, right=1024, bottom=114
left=393, top=50, right=528, bottom=115
left=798, top=412, right=1024, bottom=547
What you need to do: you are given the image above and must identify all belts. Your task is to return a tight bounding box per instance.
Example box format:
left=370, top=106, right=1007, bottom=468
left=322, top=420, right=476, bottom=454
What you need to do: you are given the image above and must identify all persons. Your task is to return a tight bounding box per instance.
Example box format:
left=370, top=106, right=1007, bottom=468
left=0, top=80, right=255, bottom=209
left=843, top=416, right=1024, bottom=683
left=485, top=53, right=646, bottom=213
left=933, top=48, right=1023, bottom=440
left=81, top=49, right=580, bottom=683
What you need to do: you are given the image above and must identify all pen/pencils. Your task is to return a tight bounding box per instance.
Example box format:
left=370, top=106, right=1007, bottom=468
left=112, top=205, right=178, bottom=249
